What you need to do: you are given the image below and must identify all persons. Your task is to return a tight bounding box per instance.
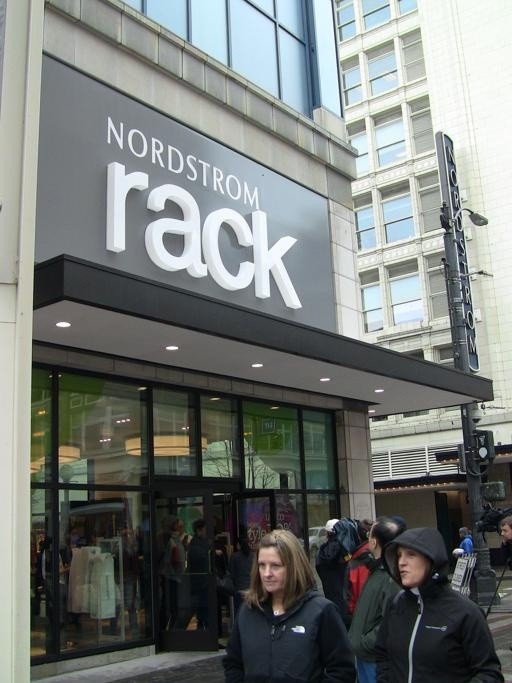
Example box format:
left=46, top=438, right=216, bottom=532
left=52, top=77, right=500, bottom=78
left=371, top=524, right=504, bottom=682
left=456, top=526, right=474, bottom=557
left=315, top=518, right=375, bottom=625
left=343, top=517, right=407, bottom=682
left=497, top=515, right=512, bottom=570
left=164, top=518, right=255, bottom=641
left=60, top=535, right=82, bottom=567
left=221, top=528, right=355, bottom=682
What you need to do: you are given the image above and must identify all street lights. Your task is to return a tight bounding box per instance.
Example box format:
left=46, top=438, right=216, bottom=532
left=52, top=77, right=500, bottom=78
left=58, top=464, right=74, bottom=556
left=437, top=198, right=503, bottom=605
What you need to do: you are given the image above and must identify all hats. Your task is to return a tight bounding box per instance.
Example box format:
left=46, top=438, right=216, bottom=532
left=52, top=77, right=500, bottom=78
left=324, top=519, right=340, bottom=533
